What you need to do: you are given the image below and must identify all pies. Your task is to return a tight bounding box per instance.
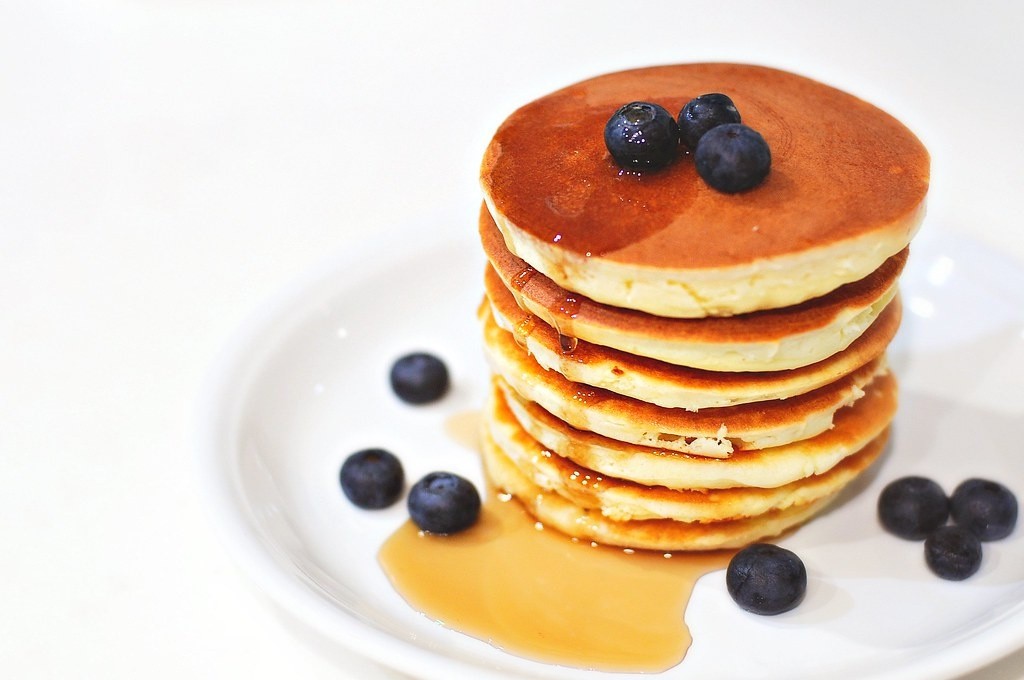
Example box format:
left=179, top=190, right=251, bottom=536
left=476, top=60, right=931, bottom=555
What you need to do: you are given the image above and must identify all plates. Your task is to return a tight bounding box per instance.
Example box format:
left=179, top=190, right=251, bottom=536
left=212, top=206, right=1024, bottom=680
